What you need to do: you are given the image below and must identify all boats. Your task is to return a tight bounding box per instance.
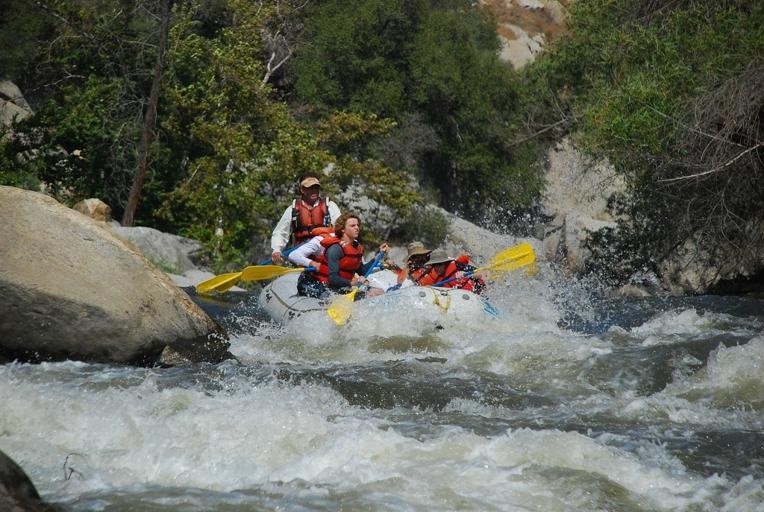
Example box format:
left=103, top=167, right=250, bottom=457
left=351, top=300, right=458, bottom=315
left=255, top=268, right=504, bottom=359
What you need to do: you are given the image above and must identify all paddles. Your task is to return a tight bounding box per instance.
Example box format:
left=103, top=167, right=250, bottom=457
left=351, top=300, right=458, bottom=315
left=432, top=242, right=535, bottom=286
left=195, top=240, right=310, bottom=297
left=241, top=265, right=317, bottom=280
left=327, top=249, right=384, bottom=325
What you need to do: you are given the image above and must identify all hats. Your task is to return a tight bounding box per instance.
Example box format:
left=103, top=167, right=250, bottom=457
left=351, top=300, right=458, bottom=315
left=300, top=175, right=320, bottom=188
left=402, top=242, right=454, bottom=265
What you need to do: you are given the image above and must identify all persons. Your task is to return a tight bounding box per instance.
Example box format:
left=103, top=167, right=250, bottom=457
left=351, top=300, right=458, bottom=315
left=271, top=175, right=488, bottom=298
